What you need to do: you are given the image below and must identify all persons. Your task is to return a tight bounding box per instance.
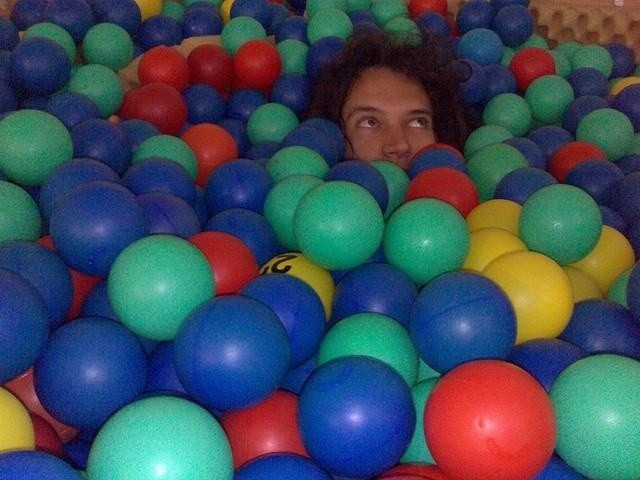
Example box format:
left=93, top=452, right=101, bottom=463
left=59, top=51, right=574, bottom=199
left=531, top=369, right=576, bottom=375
left=315, top=35, right=445, bottom=171
